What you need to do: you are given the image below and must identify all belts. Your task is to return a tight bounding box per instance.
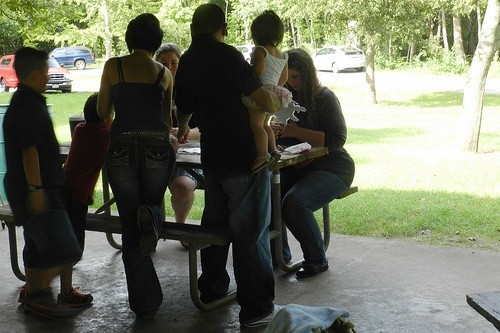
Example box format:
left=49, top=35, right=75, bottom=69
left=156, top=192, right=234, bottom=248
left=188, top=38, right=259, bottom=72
left=120, top=128, right=168, bottom=137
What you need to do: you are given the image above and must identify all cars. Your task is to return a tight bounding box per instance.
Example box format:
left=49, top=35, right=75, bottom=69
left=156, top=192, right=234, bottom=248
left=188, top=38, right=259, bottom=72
left=311, top=45, right=367, bottom=74
left=236, top=45, right=255, bottom=65
left=0, top=54, right=73, bottom=93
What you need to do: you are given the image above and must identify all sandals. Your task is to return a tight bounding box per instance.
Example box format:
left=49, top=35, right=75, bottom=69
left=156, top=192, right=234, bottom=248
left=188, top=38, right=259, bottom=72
left=268, top=150, right=281, bottom=171
left=249, top=153, right=275, bottom=176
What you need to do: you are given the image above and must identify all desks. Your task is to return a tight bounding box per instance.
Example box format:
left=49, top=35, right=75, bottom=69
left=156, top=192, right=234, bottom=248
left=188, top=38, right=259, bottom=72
left=59, top=143, right=331, bottom=312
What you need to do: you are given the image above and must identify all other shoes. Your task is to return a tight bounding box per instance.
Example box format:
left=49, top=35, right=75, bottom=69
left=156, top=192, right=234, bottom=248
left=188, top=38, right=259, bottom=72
left=181, top=240, right=189, bottom=249
left=296, top=263, right=328, bottom=279
left=137, top=206, right=157, bottom=257
left=135, top=312, right=153, bottom=321
left=23, top=291, right=77, bottom=321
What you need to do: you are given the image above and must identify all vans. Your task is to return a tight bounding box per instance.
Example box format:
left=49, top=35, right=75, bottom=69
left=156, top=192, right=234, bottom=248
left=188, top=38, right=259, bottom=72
left=49, top=46, right=96, bottom=71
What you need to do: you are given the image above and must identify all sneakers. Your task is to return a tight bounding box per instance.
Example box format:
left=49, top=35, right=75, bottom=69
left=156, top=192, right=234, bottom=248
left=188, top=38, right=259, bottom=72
left=240, top=304, right=286, bottom=328
left=18, top=288, right=28, bottom=303
left=57, top=288, right=94, bottom=308
left=201, top=299, right=213, bottom=304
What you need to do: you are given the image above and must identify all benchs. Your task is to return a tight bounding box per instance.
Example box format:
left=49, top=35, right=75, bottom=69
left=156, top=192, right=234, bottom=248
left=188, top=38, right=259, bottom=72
left=0, top=204, right=238, bottom=304
left=323, top=185, right=359, bottom=252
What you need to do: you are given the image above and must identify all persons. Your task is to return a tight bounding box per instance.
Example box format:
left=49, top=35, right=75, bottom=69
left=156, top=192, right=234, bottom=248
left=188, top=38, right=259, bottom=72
left=3, top=46, right=83, bottom=321
left=176, top=3, right=287, bottom=328
left=154, top=42, right=200, bottom=248
left=268, top=48, right=356, bottom=278
left=97, top=13, right=177, bottom=320
left=246, top=9, right=289, bottom=175
left=17, top=93, right=114, bottom=307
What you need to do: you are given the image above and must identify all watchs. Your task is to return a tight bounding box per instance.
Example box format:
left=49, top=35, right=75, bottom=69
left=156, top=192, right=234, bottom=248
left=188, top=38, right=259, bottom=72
left=27, top=184, right=42, bottom=192
